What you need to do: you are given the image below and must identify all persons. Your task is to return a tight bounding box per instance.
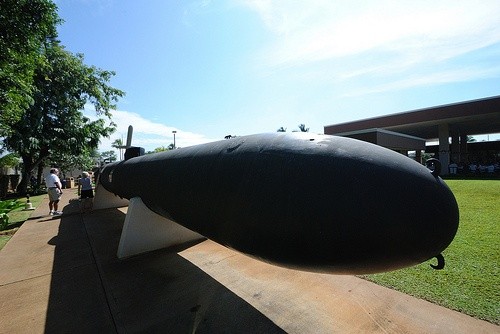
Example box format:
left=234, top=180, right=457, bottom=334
left=79, top=171, right=95, bottom=211
left=30, top=174, right=82, bottom=192
left=46, top=168, right=63, bottom=216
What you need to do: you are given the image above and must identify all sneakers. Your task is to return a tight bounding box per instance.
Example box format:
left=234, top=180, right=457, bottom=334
left=53, top=211, right=63, bottom=215
left=49, top=212, right=54, bottom=215
